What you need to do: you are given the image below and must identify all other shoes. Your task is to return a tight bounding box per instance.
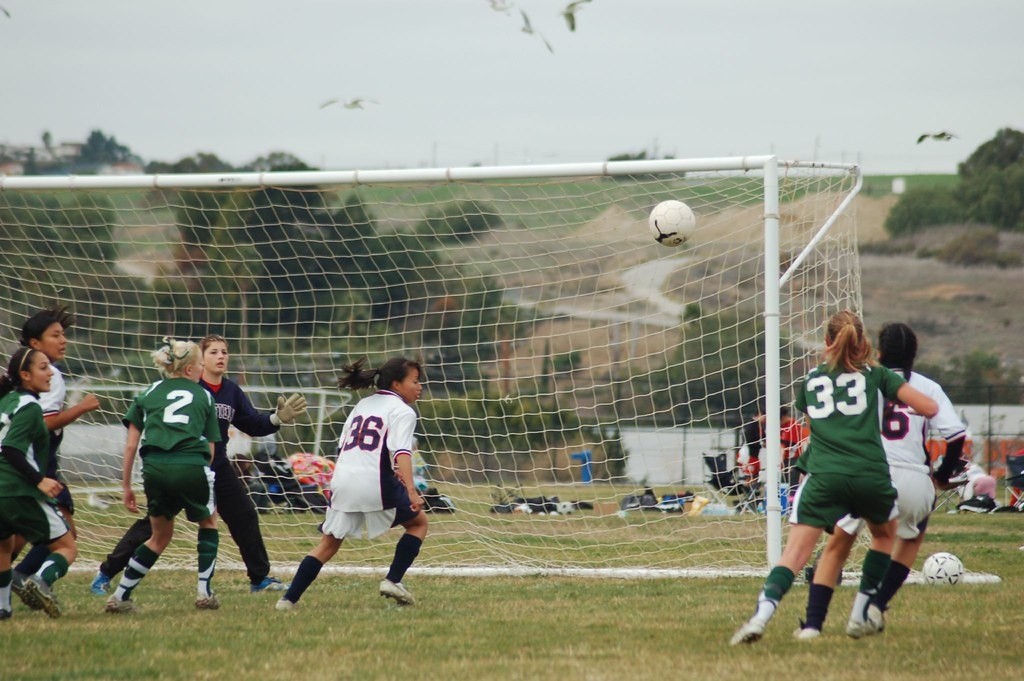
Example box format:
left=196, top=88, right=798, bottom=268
left=866, top=602, right=885, bottom=630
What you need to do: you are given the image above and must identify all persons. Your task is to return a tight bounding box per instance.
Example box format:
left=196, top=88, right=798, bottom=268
left=105, top=336, right=222, bottom=614
left=736, top=411, right=766, bottom=487
left=729, top=310, right=938, bottom=646
left=91, top=334, right=308, bottom=597
left=0, top=347, right=78, bottom=621
left=793, top=323, right=967, bottom=641
left=780, top=405, right=804, bottom=495
left=10, top=310, right=100, bottom=610
left=275, top=357, right=429, bottom=611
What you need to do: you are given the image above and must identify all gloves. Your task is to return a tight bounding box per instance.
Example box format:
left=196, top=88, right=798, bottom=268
left=270, top=392, right=307, bottom=426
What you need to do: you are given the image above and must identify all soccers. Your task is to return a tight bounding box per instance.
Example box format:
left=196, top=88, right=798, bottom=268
left=556, top=500, right=575, bottom=516
left=921, top=551, right=965, bottom=589
left=647, top=199, right=696, bottom=249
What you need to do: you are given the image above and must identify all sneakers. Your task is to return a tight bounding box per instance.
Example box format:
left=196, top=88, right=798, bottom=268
left=275, top=598, right=293, bottom=610
left=729, top=618, right=763, bottom=645
left=90, top=571, right=111, bottom=596
left=792, top=627, right=820, bottom=639
left=379, top=579, right=416, bottom=604
left=194, top=594, right=221, bottom=609
left=11, top=570, right=43, bottom=609
left=847, top=620, right=875, bottom=638
left=27, top=575, right=63, bottom=618
left=251, top=577, right=292, bottom=592
left=105, top=595, right=133, bottom=612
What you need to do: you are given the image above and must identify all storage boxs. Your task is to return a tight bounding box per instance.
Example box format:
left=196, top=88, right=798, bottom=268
left=594, top=502, right=619, bottom=515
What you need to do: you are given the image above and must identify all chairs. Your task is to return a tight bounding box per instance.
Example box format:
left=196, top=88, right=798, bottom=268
left=703, top=452, right=764, bottom=517
left=935, top=455, right=1024, bottom=508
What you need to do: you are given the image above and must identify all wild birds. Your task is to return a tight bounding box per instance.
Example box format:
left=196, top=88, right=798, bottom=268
left=317, top=99, right=380, bottom=110
left=482, top=0, right=593, bottom=53
left=915, top=131, right=960, bottom=143
